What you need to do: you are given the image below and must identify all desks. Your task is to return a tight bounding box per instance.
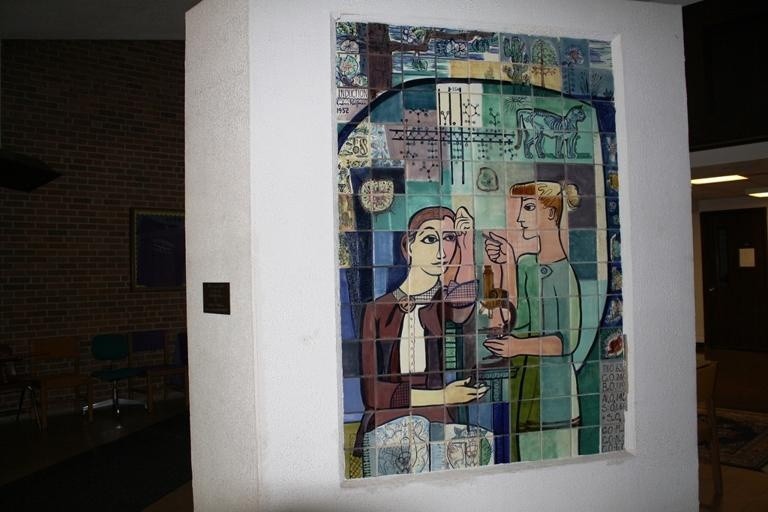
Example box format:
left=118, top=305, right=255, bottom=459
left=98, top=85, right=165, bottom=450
left=696, top=359, right=724, bottom=496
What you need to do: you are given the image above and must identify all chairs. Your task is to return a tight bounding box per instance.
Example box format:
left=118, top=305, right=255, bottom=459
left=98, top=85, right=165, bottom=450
left=2, top=330, right=189, bottom=434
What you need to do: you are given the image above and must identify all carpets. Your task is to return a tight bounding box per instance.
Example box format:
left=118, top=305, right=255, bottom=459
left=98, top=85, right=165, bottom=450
left=694, top=407, right=768, bottom=470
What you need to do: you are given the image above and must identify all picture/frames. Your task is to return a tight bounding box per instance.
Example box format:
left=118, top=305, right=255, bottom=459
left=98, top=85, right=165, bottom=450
left=128, top=206, right=184, bottom=292
